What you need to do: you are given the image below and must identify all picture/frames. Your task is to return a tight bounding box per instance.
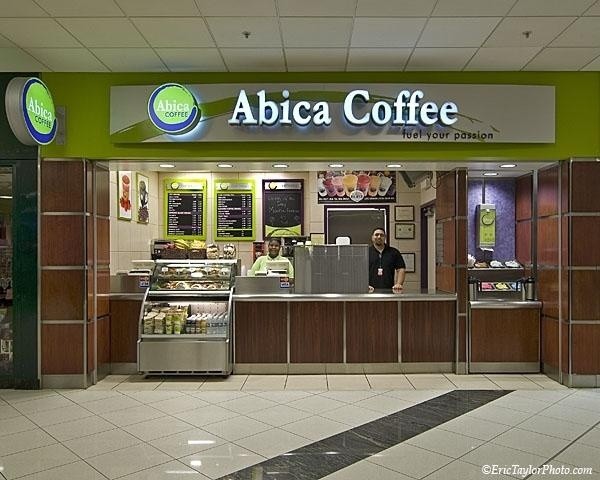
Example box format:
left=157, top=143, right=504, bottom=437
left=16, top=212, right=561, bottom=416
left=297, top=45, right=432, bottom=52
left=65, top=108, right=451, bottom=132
left=394, top=205, right=416, bottom=240
left=116, top=169, right=151, bottom=224
left=399, top=252, right=416, bottom=274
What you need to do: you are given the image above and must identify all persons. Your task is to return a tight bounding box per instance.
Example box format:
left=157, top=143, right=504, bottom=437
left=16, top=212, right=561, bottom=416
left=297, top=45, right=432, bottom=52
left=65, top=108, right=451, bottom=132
left=249, top=240, right=295, bottom=285
left=369, top=228, right=406, bottom=289
left=369, top=289, right=403, bottom=294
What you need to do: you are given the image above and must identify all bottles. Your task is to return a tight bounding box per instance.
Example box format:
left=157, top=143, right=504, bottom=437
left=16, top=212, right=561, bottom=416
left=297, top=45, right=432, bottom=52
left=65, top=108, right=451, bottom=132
left=205, top=311, right=228, bottom=335
left=515, top=275, right=536, bottom=301
left=468, top=275, right=482, bottom=301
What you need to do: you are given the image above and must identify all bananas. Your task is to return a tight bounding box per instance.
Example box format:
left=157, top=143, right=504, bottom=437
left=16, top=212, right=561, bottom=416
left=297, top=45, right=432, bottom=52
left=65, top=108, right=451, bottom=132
left=173, top=239, right=206, bottom=250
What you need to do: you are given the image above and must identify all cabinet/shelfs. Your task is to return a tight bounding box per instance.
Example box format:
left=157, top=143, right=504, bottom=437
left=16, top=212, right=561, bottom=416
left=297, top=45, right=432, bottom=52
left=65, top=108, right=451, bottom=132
left=137, top=257, right=240, bottom=377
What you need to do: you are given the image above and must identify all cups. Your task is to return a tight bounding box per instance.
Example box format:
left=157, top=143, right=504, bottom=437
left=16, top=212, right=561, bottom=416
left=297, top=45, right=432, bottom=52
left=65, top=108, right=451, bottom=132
left=315, top=172, right=392, bottom=197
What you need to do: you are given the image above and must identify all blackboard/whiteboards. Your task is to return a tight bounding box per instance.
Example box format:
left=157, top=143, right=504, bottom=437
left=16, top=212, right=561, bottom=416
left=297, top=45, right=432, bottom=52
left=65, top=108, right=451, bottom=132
left=323, top=203, right=389, bottom=247
left=262, top=190, right=305, bottom=242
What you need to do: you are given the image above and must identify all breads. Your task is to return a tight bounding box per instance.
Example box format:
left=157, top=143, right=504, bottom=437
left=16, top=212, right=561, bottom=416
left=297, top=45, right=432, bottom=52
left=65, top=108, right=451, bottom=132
left=155, top=267, right=230, bottom=290
